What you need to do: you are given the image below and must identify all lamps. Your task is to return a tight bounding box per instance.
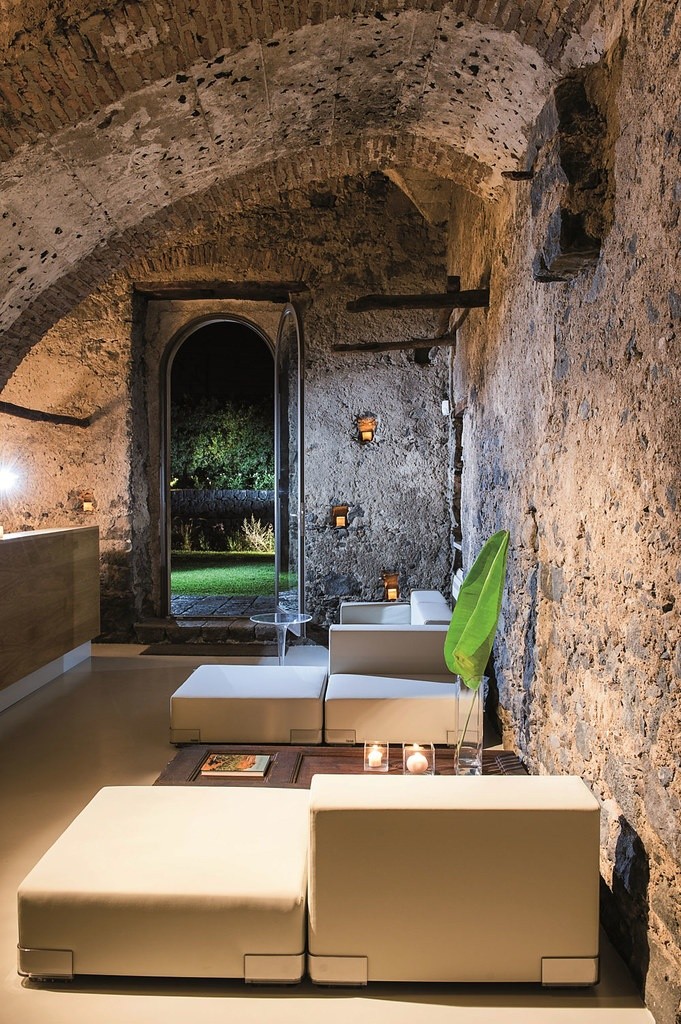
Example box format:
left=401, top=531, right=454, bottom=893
left=329, top=504, right=349, bottom=530
left=379, top=568, right=400, bottom=600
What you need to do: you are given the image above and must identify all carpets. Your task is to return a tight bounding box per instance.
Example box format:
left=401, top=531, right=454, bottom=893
left=138, top=641, right=288, bottom=657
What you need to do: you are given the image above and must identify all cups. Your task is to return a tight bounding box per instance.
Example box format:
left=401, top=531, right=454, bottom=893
left=454, top=737, right=482, bottom=778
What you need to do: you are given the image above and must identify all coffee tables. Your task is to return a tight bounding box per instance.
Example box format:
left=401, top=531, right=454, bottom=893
left=152, top=744, right=530, bottom=787
left=251, top=613, right=312, bottom=665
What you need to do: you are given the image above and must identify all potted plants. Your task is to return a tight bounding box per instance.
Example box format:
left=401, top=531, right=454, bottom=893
left=443, top=527, right=513, bottom=774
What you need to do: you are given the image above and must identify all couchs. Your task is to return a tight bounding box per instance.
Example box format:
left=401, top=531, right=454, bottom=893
left=306, top=772, right=601, bottom=988
left=16, top=785, right=304, bottom=996
left=328, top=589, right=454, bottom=675
left=167, top=664, right=328, bottom=745
left=324, top=673, right=483, bottom=744
